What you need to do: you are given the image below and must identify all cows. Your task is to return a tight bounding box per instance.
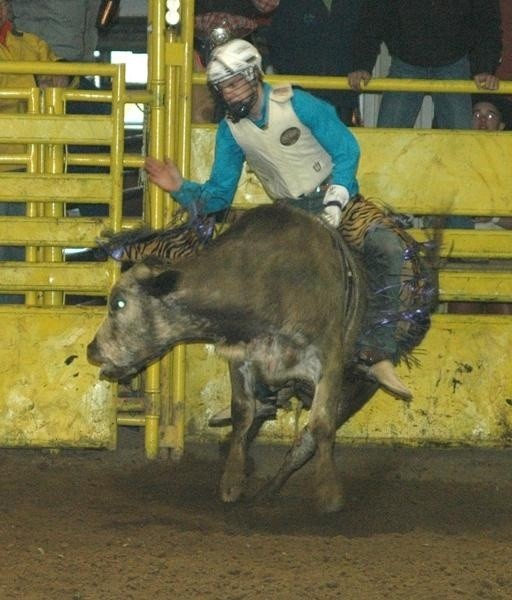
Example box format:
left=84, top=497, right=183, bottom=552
left=84, top=201, right=456, bottom=541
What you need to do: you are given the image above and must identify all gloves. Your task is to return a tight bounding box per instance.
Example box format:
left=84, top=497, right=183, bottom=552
left=319, top=184, right=350, bottom=230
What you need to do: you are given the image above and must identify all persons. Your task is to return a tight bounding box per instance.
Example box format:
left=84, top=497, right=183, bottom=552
left=143, top=35, right=416, bottom=428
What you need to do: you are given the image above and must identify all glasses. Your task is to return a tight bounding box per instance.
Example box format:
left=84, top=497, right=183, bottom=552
left=472, top=110, right=504, bottom=123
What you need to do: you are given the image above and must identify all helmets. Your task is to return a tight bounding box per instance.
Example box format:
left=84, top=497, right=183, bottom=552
left=206, top=38, right=266, bottom=91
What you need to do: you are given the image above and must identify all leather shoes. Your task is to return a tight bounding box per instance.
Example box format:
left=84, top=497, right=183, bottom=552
left=207, top=397, right=280, bottom=427
left=354, top=354, right=413, bottom=405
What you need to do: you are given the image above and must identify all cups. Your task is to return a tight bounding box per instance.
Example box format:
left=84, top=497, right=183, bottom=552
left=94, top=1, right=119, bottom=29
